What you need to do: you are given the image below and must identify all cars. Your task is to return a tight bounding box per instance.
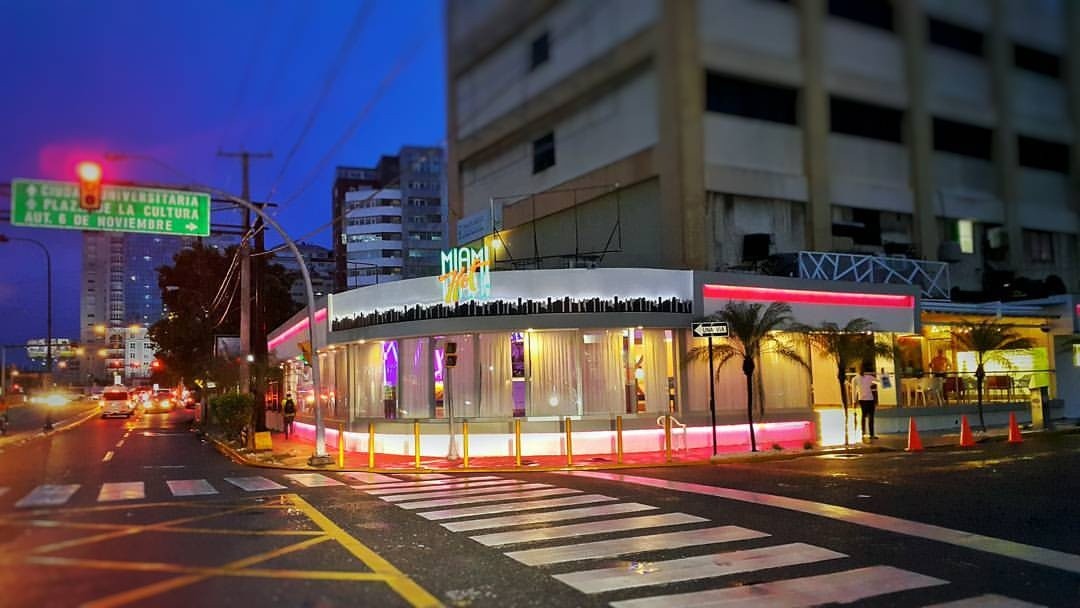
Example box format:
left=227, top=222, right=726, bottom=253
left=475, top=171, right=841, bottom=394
left=101, top=387, right=138, bottom=418
left=143, top=393, right=177, bottom=414
left=23, top=386, right=175, bottom=405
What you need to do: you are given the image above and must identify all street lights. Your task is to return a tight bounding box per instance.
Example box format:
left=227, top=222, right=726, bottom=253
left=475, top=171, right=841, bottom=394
left=0, top=235, right=56, bottom=434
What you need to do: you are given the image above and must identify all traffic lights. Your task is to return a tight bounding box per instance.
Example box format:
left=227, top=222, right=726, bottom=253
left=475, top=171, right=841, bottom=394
left=152, top=361, right=165, bottom=367
left=109, top=362, right=124, bottom=368
left=79, top=163, right=101, bottom=209
left=130, top=362, right=141, bottom=368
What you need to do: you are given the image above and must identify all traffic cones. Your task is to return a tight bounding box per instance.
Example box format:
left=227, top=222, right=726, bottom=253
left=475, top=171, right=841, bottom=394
left=1008, top=410, right=1025, bottom=444
left=904, top=415, right=928, bottom=452
left=958, top=414, right=983, bottom=451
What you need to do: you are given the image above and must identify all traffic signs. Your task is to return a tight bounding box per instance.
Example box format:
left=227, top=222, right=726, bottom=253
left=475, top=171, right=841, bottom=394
left=10, top=178, right=211, bottom=237
left=691, top=322, right=730, bottom=338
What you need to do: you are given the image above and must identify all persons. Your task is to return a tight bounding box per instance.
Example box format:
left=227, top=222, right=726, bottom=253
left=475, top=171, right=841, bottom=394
left=928, top=348, right=952, bottom=377
left=281, top=392, right=297, bottom=439
left=853, top=364, right=880, bottom=439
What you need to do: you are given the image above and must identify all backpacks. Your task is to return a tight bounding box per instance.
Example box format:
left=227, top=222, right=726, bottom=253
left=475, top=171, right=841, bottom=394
left=285, top=399, right=294, bottom=413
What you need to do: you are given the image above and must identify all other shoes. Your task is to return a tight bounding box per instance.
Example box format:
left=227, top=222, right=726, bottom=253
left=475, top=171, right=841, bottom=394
left=870, top=435, right=879, bottom=439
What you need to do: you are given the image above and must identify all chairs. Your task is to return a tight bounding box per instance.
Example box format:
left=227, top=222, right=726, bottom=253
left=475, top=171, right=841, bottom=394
left=900, top=375, right=1030, bottom=409
